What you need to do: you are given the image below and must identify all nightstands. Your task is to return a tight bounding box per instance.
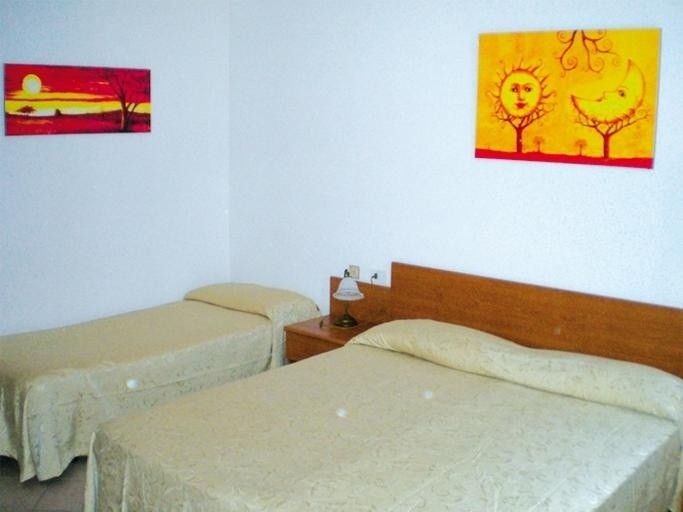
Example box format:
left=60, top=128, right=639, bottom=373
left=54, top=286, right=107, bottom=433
left=283, top=276, right=390, bottom=365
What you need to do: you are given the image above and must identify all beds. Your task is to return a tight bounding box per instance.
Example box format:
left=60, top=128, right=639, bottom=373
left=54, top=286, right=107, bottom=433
left=0, top=281, right=322, bottom=482
left=86, top=260, right=683, bottom=512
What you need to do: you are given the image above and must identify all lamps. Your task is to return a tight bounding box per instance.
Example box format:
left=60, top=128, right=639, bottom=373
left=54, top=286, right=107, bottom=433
left=332, top=269, right=365, bottom=326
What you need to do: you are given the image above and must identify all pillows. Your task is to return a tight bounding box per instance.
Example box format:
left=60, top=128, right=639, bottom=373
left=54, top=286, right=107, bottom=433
left=183, top=280, right=315, bottom=324
left=347, top=318, right=683, bottom=424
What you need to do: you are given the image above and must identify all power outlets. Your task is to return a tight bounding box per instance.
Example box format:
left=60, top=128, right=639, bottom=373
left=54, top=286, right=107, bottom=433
left=349, top=265, right=385, bottom=282
